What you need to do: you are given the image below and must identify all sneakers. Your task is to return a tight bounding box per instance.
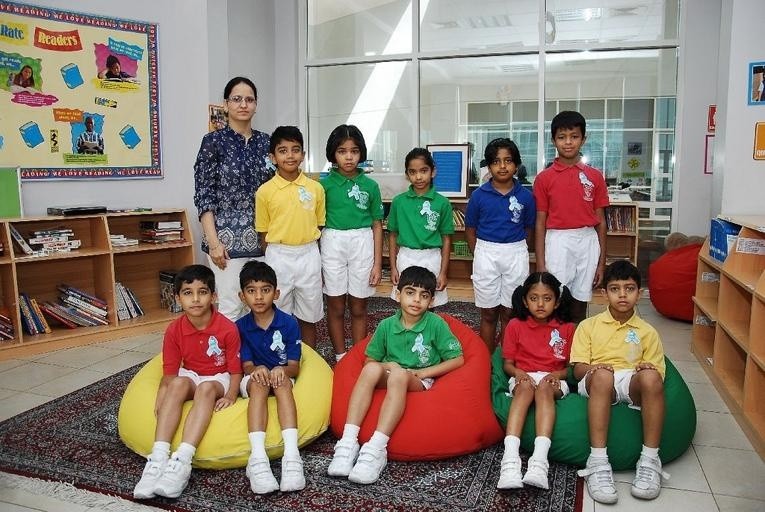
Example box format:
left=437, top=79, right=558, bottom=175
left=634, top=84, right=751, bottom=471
left=279, top=449, right=306, bottom=493
left=522, top=455, right=550, bottom=490
left=577, top=453, right=618, bottom=504
left=327, top=438, right=387, bottom=484
left=134, top=453, right=192, bottom=500
left=245, top=453, right=279, bottom=494
left=497, top=455, right=524, bottom=489
left=631, top=455, right=671, bottom=500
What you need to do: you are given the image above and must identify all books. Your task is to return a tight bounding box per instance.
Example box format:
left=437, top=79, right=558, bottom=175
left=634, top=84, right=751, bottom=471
left=0, top=207, right=187, bottom=340
left=380, top=208, right=465, bottom=282
left=607, top=206, right=635, bottom=233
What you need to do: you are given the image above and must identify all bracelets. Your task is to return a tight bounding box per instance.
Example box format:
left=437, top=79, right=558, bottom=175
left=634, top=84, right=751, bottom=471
left=208, top=240, right=221, bottom=252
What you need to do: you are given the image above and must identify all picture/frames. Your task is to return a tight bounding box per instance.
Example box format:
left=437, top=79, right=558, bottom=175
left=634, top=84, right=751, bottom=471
left=704, top=134, right=715, bottom=175
left=746, top=61, right=764, bottom=106
left=424, top=142, right=472, bottom=200
left=0, top=0, right=165, bottom=181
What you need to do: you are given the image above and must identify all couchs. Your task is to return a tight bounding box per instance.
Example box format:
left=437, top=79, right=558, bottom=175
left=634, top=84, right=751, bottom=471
left=115, top=331, right=334, bottom=472
left=646, top=243, right=702, bottom=321
left=332, top=310, right=504, bottom=464
left=489, top=333, right=700, bottom=466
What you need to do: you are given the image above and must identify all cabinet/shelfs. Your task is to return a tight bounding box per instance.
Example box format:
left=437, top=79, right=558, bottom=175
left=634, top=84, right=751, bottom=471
left=369, top=197, right=639, bottom=305
left=92, top=208, right=197, bottom=337
left=689, top=212, right=765, bottom=462
left=0, top=217, right=25, bottom=360
left=4, top=210, right=118, bottom=353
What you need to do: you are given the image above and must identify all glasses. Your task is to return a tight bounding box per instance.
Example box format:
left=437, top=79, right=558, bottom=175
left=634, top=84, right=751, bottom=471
left=226, top=95, right=257, bottom=105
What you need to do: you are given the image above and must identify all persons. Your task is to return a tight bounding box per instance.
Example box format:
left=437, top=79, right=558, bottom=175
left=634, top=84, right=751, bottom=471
left=235, top=259, right=307, bottom=495
left=532, top=110, right=610, bottom=322
left=569, top=260, right=671, bottom=505
left=75, top=116, right=104, bottom=154
left=318, top=125, right=385, bottom=365
left=756, top=72, right=765, bottom=101
left=14, top=65, right=34, bottom=87
left=193, top=77, right=273, bottom=323
left=326, top=266, right=464, bottom=485
left=133, top=264, right=244, bottom=499
left=254, top=125, right=326, bottom=350
left=387, top=147, right=455, bottom=312
left=98, top=54, right=135, bottom=82
left=497, top=272, right=576, bottom=491
left=464, top=138, right=537, bottom=354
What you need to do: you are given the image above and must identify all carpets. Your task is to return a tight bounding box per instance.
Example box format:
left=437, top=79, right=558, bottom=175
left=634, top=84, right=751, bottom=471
left=1, top=293, right=588, bottom=512
